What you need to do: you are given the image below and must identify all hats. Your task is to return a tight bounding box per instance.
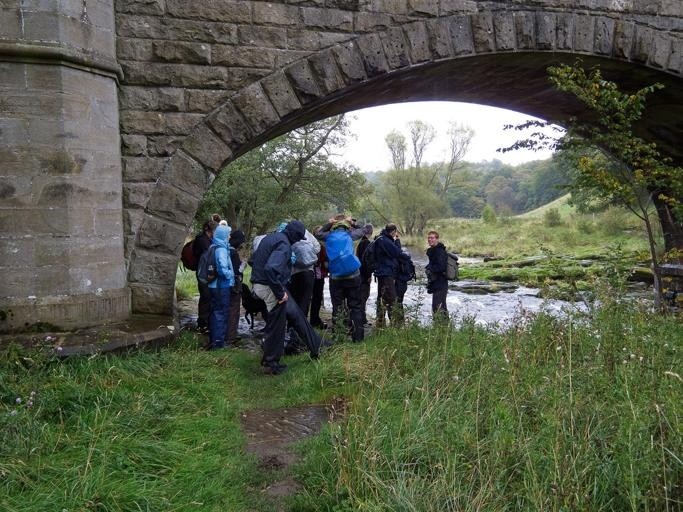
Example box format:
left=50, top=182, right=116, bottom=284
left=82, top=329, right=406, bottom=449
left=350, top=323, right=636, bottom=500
left=286, top=220, right=307, bottom=244
left=331, top=215, right=351, bottom=229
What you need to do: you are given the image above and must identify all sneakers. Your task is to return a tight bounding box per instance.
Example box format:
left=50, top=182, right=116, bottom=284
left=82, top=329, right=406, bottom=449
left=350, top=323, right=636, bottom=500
left=252, top=320, right=371, bottom=375
left=197, top=325, right=241, bottom=350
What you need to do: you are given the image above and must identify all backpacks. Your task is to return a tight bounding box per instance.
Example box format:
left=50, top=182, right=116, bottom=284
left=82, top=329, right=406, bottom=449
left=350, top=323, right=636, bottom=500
left=182, top=242, right=458, bottom=286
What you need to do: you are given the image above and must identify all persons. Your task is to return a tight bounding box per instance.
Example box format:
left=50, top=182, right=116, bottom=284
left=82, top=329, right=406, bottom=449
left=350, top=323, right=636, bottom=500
left=426, top=231, right=448, bottom=322
left=194, top=215, right=410, bottom=377
